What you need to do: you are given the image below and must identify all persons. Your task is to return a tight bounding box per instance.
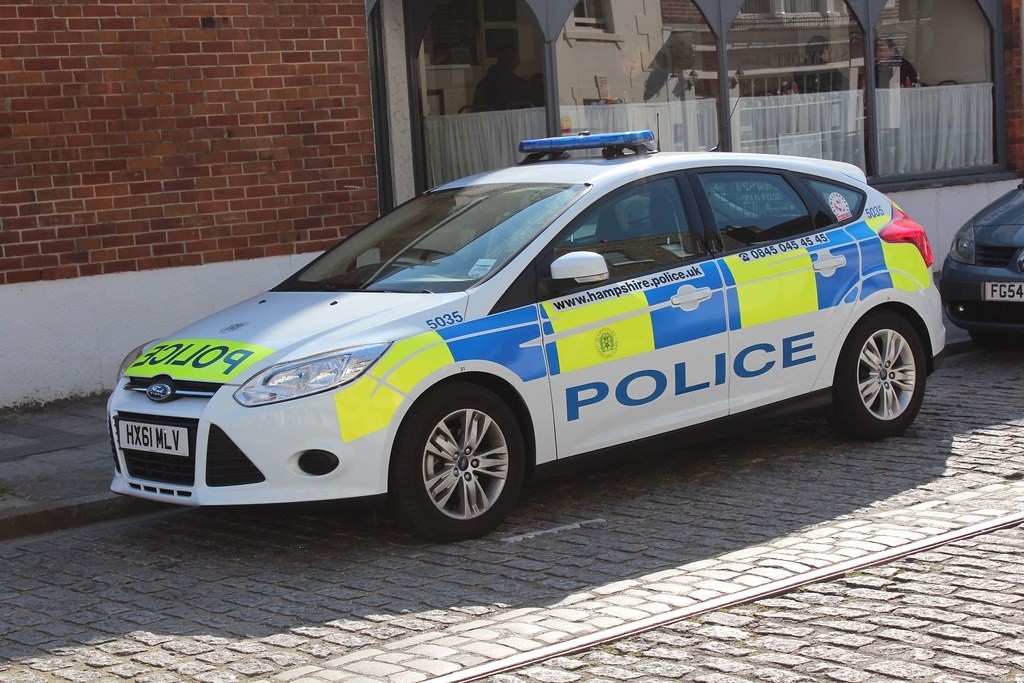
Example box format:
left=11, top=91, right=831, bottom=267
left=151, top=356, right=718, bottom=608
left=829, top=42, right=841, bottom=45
left=793, top=36, right=843, bottom=94
left=874, top=37, right=917, bottom=89
left=473, top=50, right=527, bottom=112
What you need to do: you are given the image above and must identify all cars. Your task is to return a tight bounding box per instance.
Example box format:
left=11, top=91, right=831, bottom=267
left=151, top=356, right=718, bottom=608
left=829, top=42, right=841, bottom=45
left=939, top=181, right=1024, bottom=354
left=104, top=127, right=948, bottom=545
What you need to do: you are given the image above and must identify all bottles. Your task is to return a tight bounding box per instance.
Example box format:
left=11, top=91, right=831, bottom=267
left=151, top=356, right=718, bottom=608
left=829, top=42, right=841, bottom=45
left=606, top=94, right=612, bottom=104
left=772, top=80, right=802, bottom=95
left=901, top=76, right=921, bottom=88
